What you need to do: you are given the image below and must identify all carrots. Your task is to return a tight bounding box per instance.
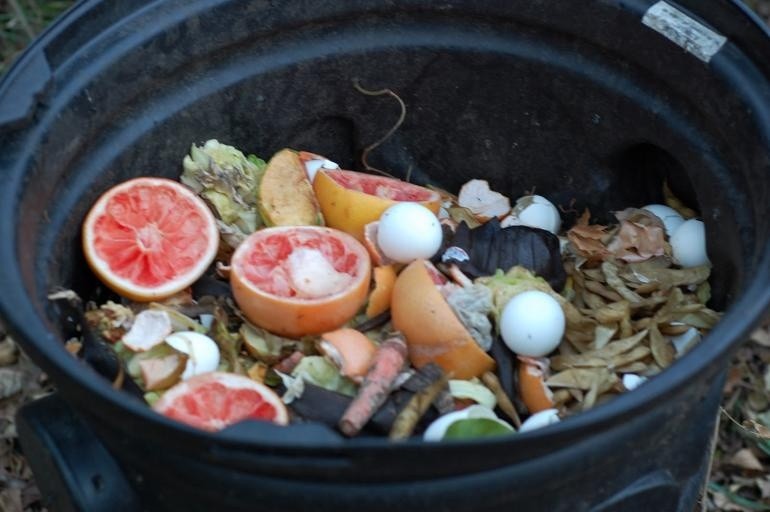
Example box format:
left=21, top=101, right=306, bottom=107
left=339, top=332, right=406, bottom=438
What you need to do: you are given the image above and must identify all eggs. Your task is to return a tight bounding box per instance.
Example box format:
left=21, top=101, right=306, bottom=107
left=374, top=202, right=443, bottom=265
left=499, top=290, right=564, bottom=358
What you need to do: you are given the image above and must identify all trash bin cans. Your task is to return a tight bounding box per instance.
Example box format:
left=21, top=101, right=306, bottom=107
left=0, top=0, right=769, bottom=512
